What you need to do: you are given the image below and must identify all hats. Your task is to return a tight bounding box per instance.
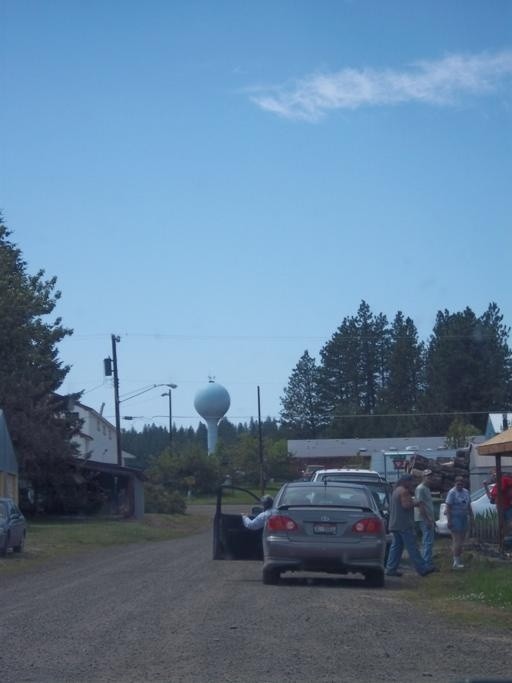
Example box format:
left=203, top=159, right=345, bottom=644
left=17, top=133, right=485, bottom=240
left=433, top=481, right=497, bottom=537
left=0, top=496, right=28, bottom=556
left=297, top=463, right=391, bottom=535
left=211, top=477, right=392, bottom=589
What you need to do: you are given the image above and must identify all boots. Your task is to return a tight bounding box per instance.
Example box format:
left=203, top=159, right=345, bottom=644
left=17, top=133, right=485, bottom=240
left=322, top=476, right=396, bottom=563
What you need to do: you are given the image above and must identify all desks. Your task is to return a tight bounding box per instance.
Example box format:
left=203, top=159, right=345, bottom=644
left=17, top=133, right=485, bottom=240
left=111, top=332, right=179, bottom=465
left=160, top=386, right=173, bottom=458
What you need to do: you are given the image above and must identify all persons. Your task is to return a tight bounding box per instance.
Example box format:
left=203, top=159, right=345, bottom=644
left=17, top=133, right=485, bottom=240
left=444, top=475, right=474, bottom=568
left=240, top=494, right=274, bottom=531
left=385, top=473, right=435, bottom=576
left=415, top=468, right=441, bottom=572
left=483, top=468, right=512, bottom=552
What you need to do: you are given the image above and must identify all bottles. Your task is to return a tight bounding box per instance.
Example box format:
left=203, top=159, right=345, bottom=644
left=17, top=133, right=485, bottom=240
left=423, top=469, right=434, bottom=476
